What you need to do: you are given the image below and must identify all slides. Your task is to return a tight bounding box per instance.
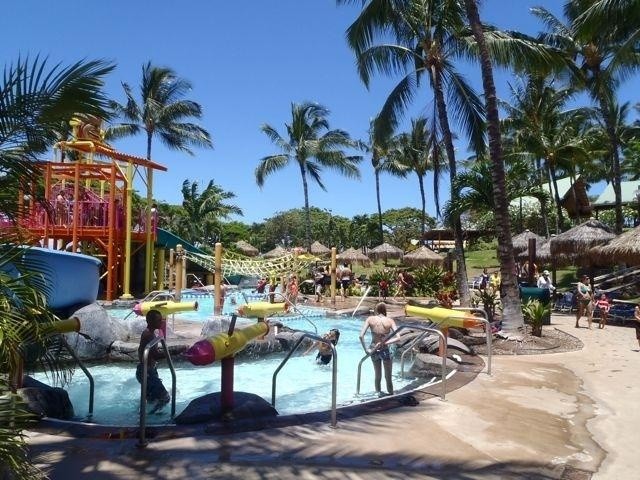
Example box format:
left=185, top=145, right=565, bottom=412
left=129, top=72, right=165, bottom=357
left=154, top=227, right=242, bottom=285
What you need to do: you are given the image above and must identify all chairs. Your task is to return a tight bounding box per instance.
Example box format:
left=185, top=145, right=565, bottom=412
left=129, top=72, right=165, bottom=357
left=555, top=292, right=636, bottom=324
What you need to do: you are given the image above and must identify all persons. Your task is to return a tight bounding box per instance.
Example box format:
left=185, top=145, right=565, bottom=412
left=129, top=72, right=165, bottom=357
left=269, top=282, right=278, bottom=303
left=394, top=269, right=409, bottom=300
left=575, top=274, right=594, bottom=328
left=597, top=293, right=611, bottom=328
left=538, top=270, right=556, bottom=290
left=288, top=276, right=298, bottom=311
left=303, top=328, right=341, bottom=365
left=336, top=262, right=351, bottom=301
left=256, top=280, right=266, bottom=294
left=480, top=270, right=501, bottom=290
left=359, top=302, right=402, bottom=395
left=634, top=305, right=640, bottom=347
left=315, top=265, right=331, bottom=303
left=220, top=280, right=226, bottom=313
left=380, top=278, right=388, bottom=300
left=135, top=309, right=170, bottom=413
left=56, top=189, right=160, bottom=233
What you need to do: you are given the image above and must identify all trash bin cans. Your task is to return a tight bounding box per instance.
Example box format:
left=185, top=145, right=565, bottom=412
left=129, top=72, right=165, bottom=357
left=520, top=287, right=550, bottom=325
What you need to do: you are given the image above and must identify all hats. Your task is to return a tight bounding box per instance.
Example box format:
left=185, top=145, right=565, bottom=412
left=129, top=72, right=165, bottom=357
left=543, top=270, right=550, bottom=275
